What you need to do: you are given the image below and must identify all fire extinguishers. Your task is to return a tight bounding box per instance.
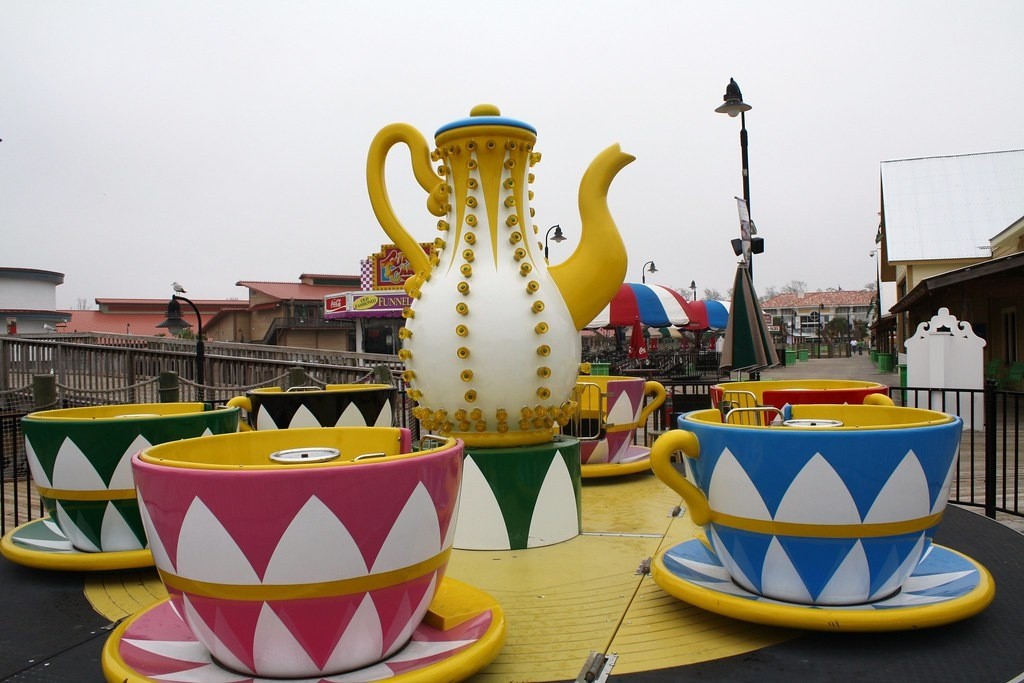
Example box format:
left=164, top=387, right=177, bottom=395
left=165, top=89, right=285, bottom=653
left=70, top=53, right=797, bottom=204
left=665, top=391, right=673, bottom=427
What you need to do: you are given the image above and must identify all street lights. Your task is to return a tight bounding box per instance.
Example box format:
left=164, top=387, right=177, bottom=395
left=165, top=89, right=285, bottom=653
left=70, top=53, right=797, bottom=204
left=543, top=224, right=567, bottom=265
left=714, top=77, right=766, bottom=280
left=155, top=294, right=206, bottom=405
left=642, top=260, right=656, bottom=285
left=689, top=281, right=696, bottom=302
left=817, top=303, right=824, bottom=359
left=870, top=249, right=881, bottom=354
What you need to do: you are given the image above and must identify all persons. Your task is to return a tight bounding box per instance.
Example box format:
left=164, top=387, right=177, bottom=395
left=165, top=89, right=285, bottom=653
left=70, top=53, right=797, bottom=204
left=850, top=338, right=858, bottom=355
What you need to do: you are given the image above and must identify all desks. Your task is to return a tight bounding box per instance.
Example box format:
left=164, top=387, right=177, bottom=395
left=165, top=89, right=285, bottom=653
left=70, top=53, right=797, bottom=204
left=621, top=369, right=660, bottom=380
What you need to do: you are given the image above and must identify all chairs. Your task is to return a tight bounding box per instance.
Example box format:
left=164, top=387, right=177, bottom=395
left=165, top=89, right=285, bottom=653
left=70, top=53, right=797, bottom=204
left=985, top=358, right=1024, bottom=392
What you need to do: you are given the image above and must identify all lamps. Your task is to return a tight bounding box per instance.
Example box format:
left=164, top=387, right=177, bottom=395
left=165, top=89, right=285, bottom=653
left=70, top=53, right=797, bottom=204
left=875, top=222, right=883, bottom=243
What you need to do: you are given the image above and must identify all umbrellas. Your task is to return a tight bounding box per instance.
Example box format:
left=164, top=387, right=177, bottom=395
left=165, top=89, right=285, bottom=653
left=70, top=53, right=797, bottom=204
left=627, top=316, right=648, bottom=360
left=584, top=282, right=702, bottom=328
left=719, top=259, right=780, bottom=380
left=671, top=300, right=731, bottom=329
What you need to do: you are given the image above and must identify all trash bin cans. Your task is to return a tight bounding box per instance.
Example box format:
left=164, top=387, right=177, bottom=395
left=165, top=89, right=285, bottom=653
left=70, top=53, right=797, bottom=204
left=786, top=351, right=796, bottom=365
left=870, top=350, right=878, bottom=361
left=897, top=364, right=908, bottom=401
left=878, top=353, right=893, bottom=373
left=590, top=363, right=611, bottom=376
left=798, top=349, right=809, bottom=362
